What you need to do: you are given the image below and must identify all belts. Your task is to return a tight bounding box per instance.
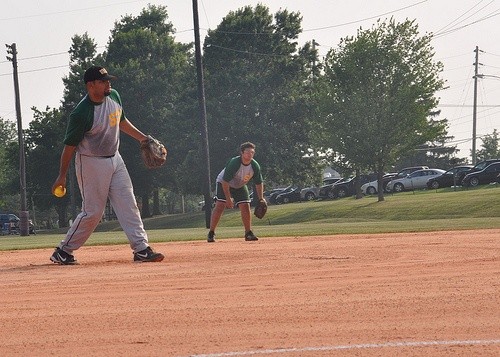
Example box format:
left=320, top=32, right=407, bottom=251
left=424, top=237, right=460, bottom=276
left=104, top=154, right=115, bottom=158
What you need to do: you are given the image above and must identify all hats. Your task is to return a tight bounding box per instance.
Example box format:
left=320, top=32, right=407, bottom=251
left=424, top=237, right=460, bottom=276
left=84, top=66, right=116, bottom=83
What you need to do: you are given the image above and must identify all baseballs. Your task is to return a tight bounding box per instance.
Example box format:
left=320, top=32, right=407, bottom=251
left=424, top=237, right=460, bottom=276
left=54, top=185, right=66, bottom=197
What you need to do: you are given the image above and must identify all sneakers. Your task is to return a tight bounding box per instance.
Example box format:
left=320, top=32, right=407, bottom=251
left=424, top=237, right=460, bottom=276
left=50, top=246, right=79, bottom=265
left=134, top=248, right=164, bottom=262
left=208, top=232, right=214, bottom=242
left=244, top=232, right=258, bottom=240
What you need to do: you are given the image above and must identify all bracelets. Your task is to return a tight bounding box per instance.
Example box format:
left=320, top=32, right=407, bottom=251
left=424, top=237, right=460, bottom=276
left=259, top=199, right=265, bottom=202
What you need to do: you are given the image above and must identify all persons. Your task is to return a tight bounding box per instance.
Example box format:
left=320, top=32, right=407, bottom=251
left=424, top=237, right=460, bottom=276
left=8, top=220, right=17, bottom=234
left=207, top=141, right=267, bottom=242
left=50, top=65, right=166, bottom=265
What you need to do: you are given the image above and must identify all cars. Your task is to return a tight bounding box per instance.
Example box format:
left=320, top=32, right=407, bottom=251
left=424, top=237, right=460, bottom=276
left=332, top=170, right=385, bottom=197
left=426, top=165, right=473, bottom=190
left=0, top=214, right=33, bottom=233
left=462, top=161, right=499, bottom=189
left=386, top=167, right=450, bottom=193
left=383, top=166, right=429, bottom=189
left=199, top=175, right=353, bottom=213
left=360, top=173, right=402, bottom=195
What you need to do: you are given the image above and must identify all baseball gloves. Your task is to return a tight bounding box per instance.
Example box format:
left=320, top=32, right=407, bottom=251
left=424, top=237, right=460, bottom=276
left=141, top=135, right=167, bottom=169
left=254, top=199, right=267, bottom=219
